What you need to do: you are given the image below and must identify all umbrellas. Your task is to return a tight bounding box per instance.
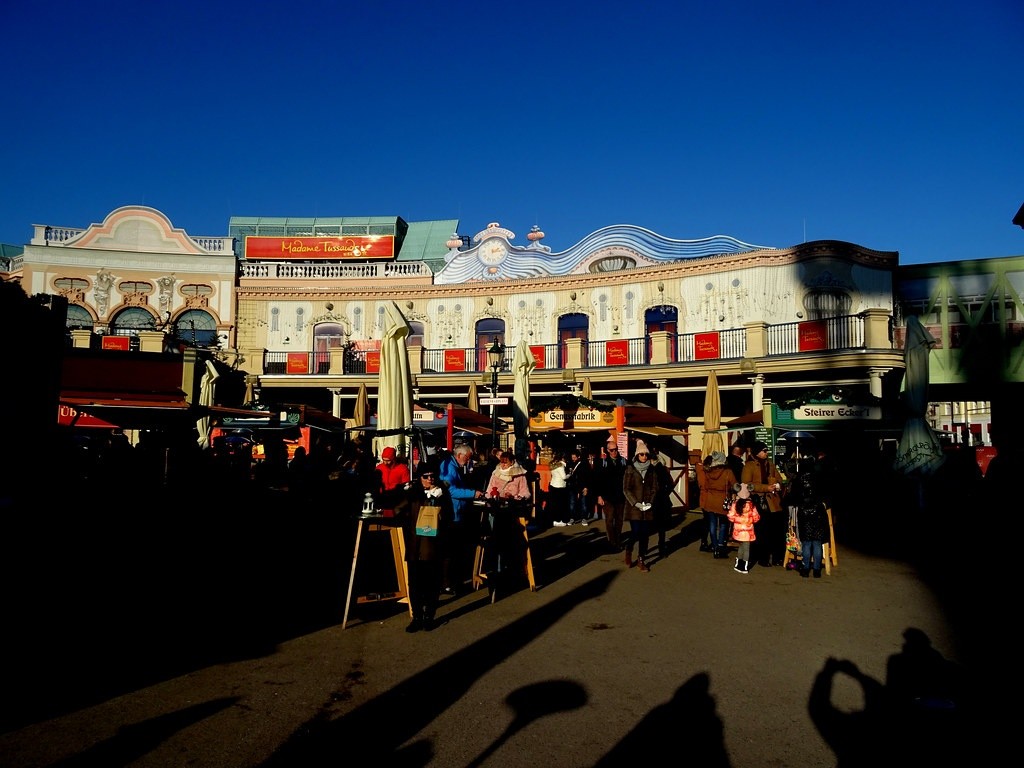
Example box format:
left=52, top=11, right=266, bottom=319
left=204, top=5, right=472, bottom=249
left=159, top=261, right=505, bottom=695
left=896, top=313, right=948, bottom=476
left=467, top=379, right=482, bottom=415
left=780, top=430, right=815, bottom=472
left=242, top=382, right=255, bottom=406
left=511, top=339, right=536, bottom=462
left=701, top=368, right=725, bottom=463
left=583, top=376, right=592, bottom=400
left=196, top=360, right=219, bottom=449
left=353, top=381, right=370, bottom=437
left=377, top=298, right=411, bottom=461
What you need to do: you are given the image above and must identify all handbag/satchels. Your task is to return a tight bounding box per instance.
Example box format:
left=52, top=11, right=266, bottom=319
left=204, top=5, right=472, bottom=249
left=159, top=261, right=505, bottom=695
left=723, top=498, right=733, bottom=510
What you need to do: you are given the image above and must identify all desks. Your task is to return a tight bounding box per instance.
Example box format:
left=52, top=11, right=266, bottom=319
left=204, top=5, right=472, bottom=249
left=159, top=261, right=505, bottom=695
left=341, top=509, right=412, bottom=630
left=785, top=505, right=838, bottom=576
left=472, top=498, right=537, bottom=591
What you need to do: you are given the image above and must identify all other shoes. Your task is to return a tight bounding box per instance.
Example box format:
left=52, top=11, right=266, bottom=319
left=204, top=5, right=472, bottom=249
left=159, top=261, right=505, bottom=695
left=774, top=558, right=782, bottom=566
left=423, top=618, right=434, bottom=631
left=567, top=519, right=575, bottom=525
left=758, top=560, right=771, bottom=567
left=406, top=618, right=423, bottom=632
left=582, top=520, right=588, bottom=526
left=813, top=569, right=821, bottom=578
left=553, top=521, right=567, bottom=527
left=700, top=541, right=713, bottom=552
left=801, top=568, right=810, bottom=576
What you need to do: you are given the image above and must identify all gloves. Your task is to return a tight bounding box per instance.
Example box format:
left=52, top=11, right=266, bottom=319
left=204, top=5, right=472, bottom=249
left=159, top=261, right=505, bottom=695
left=635, top=502, right=652, bottom=511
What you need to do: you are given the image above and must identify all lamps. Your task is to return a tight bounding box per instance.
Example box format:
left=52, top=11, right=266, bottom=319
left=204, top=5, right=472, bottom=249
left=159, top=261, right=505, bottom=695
left=657, top=282, right=664, bottom=291
left=324, top=302, right=336, bottom=312
left=568, top=291, right=577, bottom=301
left=405, top=300, right=414, bottom=310
left=486, top=295, right=494, bottom=306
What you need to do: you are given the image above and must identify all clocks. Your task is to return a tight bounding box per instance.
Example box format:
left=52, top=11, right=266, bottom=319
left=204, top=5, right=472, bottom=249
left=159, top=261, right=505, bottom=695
left=477, top=238, right=508, bottom=264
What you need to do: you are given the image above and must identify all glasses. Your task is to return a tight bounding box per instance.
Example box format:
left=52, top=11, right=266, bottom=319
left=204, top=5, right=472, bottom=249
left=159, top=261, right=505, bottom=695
left=609, top=448, right=618, bottom=452
left=422, top=474, right=434, bottom=479
left=640, top=453, right=648, bottom=456
left=762, top=449, right=768, bottom=453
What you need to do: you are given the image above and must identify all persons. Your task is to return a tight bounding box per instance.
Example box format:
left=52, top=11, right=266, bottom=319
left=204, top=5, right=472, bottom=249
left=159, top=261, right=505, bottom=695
left=439, top=444, right=484, bottom=595
left=622, top=437, right=676, bottom=572
left=725, top=446, right=744, bottom=485
left=215, top=435, right=330, bottom=480
left=703, top=450, right=738, bottom=559
left=86, top=427, right=148, bottom=456
left=785, top=458, right=830, bottom=578
left=741, top=441, right=783, bottom=567
left=593, top=440, right=627, bottom=550
left=393, top=460, right=455, bottom=633
left=374, top=447, right=410, bottom=513
left=741, top=442, right=751, bottom=465
left=485, top=447, right=531, bottom=500
left=727, top=483, right=761, bottom=574
left=550, top=449, right=589, bottom=526
left=696, top=455, right=714, bottom=552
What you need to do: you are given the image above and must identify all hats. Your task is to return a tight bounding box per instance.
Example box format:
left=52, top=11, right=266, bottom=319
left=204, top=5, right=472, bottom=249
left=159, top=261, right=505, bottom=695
left=734, top=482, right=753, bottom=499
left=712, top=451, right=727, bottom=463
left=634, top=439, right=650, bottom=455
left=382, top=447, right=396, bottom=462
left=751, top=441, right=766, bottom=455
left=417, top=461, right=435, bottom=477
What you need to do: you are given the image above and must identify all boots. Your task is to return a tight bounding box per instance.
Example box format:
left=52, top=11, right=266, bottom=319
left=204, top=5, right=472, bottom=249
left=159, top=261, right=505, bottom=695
left=638, top=555, right=649, bottom=573
left=714, top=545, right=729, bottom=559
left=625, top=551, right=634, bottom=568
left=741, top=561, right=749, bottom=573
left=734, top=557, right=743, bottom=571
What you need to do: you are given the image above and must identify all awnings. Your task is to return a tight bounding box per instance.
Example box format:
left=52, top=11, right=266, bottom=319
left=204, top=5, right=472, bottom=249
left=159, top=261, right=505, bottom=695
left=624, top=423, right=691, bottom=436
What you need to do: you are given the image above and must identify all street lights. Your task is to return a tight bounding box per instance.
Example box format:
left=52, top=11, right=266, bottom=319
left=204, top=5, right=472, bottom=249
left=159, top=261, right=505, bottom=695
left=486, top=335, right=504, bottom=448
